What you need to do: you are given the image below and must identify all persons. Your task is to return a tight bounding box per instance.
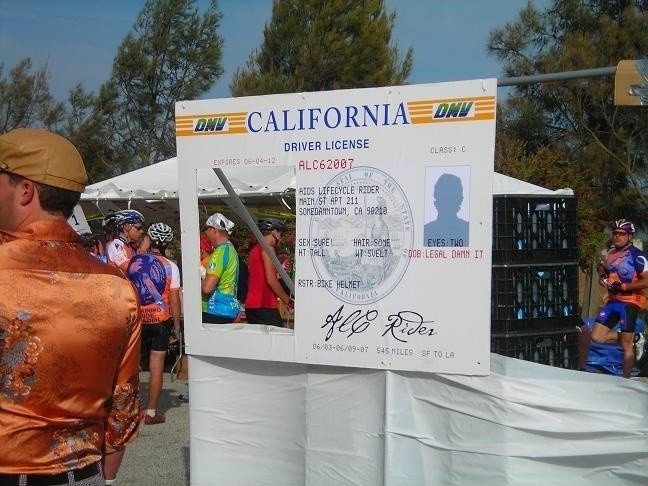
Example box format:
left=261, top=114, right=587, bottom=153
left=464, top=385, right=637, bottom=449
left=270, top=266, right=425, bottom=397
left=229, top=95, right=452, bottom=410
left=200, top=213, right=291, bottom=328
left=1, top=131, right=181, bottom=486
left=577, top=218, right=648, bottom=379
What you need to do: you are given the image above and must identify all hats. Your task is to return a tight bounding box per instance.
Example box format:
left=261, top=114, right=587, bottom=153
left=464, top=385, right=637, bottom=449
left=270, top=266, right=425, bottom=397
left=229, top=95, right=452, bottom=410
left=0, top=127, right=89, bottom=194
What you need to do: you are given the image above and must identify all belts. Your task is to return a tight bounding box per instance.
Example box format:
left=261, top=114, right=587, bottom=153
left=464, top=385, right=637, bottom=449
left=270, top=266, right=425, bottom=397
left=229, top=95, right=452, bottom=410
left=0, top=461, right=100, bottom=486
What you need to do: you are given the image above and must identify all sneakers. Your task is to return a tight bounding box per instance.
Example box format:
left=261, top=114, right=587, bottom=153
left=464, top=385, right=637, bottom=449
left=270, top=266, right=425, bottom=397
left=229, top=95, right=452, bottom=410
left=634, top=332, right=646, bottom=362
left=144, top=412, right=167, bottom=424
left=177, top=394, right=189, bottom=402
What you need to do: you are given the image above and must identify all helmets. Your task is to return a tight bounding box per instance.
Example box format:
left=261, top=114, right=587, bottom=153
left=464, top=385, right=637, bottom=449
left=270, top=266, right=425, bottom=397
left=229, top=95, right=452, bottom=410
left=257, top=217, right=287, bottom=235
left=611, top=218, right=636, bottom=234
left=113, top=209, right=146, bottom=226
left=147, top=222, right=174, bottom=246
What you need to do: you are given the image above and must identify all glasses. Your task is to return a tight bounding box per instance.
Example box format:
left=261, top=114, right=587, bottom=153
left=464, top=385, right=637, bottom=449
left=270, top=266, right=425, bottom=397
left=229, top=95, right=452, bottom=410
left=612, top=230, right=629, bottom=237
left=134, top=225, right=142, bottom=232
